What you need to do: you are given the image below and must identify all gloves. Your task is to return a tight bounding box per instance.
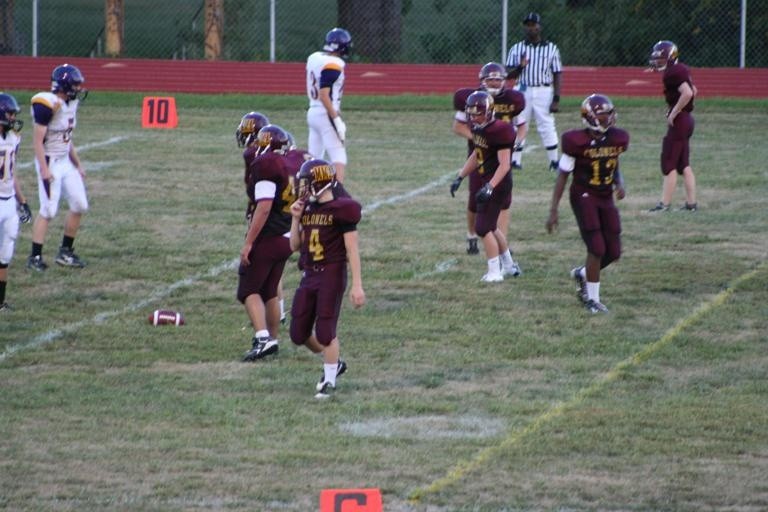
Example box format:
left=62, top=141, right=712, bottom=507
left=449, top=176, right=463, bottom=198
left=17, top=197, right=32, bottom=225
left=473, top=182, right=494, bottom=205
left=331, top=112, right=348, bottom=143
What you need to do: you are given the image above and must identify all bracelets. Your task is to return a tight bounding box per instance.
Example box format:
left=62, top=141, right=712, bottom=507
left=552, top=94, right=560, bottom=102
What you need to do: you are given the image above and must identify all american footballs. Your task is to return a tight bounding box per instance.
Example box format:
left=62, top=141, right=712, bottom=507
left=149, top=309, right=184, bottom=325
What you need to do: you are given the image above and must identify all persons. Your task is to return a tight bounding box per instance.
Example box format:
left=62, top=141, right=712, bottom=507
left=0, top=92, right=34, bottom=312
left=25, top=62, right=91, bottom=272
left=234, top=111, right=366, bottom=402
left=501, top=11, right=565, bottom=171
left=544, top=92, right=631, bottom=314
left=637, top=37, right=699, bottom=215
left=305, top=27, right=356, bottom=186
left=566, top=0, right=768, bottom=70
left=449, top=89, right=522, bottom=283
left=449, top=61, right=526, bottom=255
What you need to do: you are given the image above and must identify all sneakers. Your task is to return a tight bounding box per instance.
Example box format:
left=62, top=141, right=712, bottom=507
left=465, top=233, right=480, bottom=256
left=53, top=247, right=87, bottom=270
left=569, top=264, right=589, bottom=303
left=641, top=200, right=672, bottom=216
left=547, top=159, right=558, bottom=173
left=242, top=336, right=280, bottom=363
left=511, top=161, right=523, bottom=170
left=500, top=261, right=521, bottom=279
left=583, top=298, right=611, bottom=316
left=313, top=380, right=336, bottom=400
left=279, top=316, right=288, bottom=328
left=27, top=255, right=49, bottom=273
left=673, top=200, right=698, bottom=214
left=314, top=356, right=349, bottom=391
left=0, top=302, right=15, bottom=313
left=473, top=274, right=505, bottom=286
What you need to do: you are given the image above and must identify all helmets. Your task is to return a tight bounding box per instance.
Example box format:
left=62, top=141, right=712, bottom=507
left=0, top=92, right=24, bottom=132
left=50, top=64, right=85, bottom=101
left=579, top=93, right=617, bottom=132
left=251, top=124, right=289, bottom=158
left=291, top=158, right=337, bottom=203
left=463, top=90, right=495, bottom=132
left=478, top=61, right=508, bottom=97
left=322, top=27, right=354, bottom=60
left=236, top=112, right=269, bottom=149
left=286, top=131, right=297, bottom=151
left=648, top=40, right=679, bottom=73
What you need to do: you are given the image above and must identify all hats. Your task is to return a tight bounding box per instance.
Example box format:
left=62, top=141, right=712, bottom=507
left=521, top=12, right=542, bottom=25
